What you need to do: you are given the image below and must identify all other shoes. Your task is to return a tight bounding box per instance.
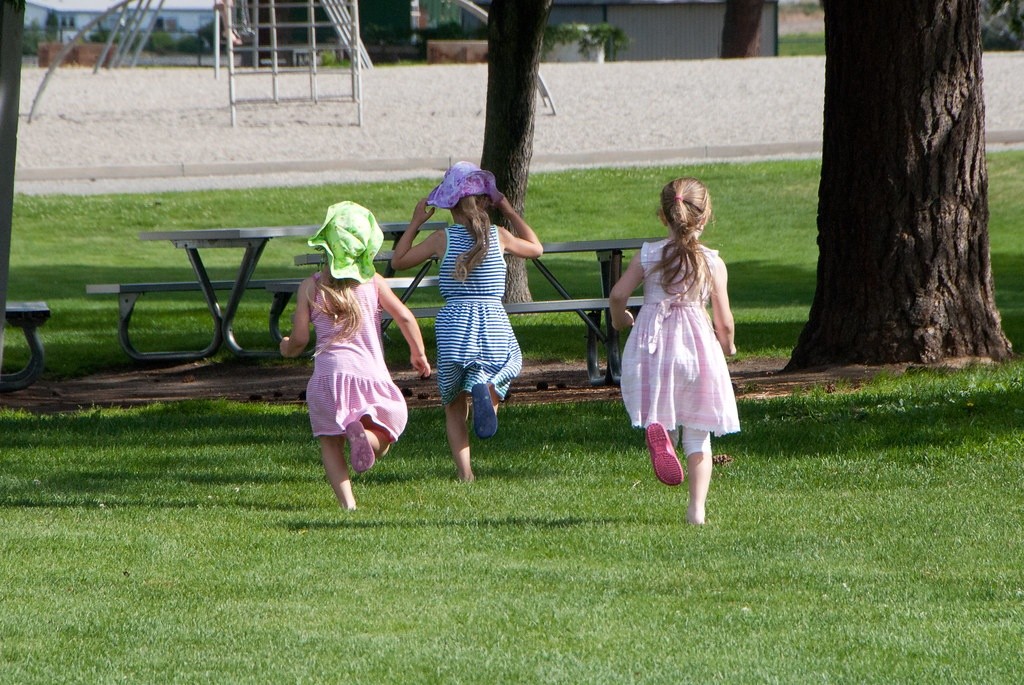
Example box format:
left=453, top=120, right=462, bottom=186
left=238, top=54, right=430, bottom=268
left=472, top=383, right=497, bottom=438
left=346, top=421, right=375, bottom=473
left=646, top=423, right=683, bottom=485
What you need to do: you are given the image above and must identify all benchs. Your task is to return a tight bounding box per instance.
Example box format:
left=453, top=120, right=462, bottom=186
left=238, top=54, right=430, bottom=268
left=86, top=276, right=308, bottom=361
left=0, top=301, right=51, bottom=395
left=382, top=295, right=646, bottom=388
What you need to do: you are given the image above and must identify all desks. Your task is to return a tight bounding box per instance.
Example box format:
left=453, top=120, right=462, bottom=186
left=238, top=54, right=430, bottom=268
left=139, top=220, right=449, bottom=360
left=501, top=234, right=670, bottom=388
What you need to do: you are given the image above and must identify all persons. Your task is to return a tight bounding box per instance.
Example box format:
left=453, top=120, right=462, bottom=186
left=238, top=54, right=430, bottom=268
left=384, top=159, right=546, bottom=483
left=277, top=196, right=437, bottom=518
left=607, top=174, right=740, bottom=525
